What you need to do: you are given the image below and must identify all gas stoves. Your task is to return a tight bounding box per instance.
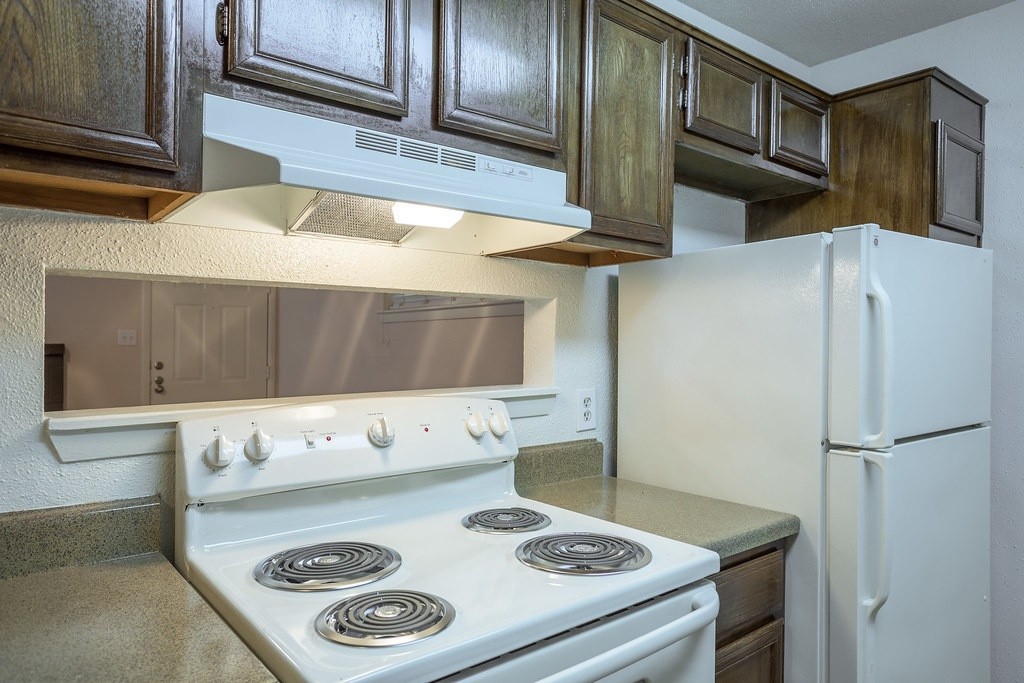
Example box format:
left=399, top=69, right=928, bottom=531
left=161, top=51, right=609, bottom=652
left=174, top=395, right=719, bottom=683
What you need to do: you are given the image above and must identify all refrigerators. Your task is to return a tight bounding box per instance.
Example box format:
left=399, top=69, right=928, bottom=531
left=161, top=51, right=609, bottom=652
left=618, top=223, right=992, bottom=683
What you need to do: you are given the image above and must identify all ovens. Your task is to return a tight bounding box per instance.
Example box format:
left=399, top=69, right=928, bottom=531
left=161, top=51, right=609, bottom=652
left=435, top=582, right=719, bottom=683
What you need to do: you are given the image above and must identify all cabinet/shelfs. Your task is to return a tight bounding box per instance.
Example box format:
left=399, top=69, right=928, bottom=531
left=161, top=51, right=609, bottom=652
left=484, top=0, right=676, bottom=269
left=0, top=0, right=201, bottom=226
left=676, top=16, right=833, bottom=205
left=44, top=343, right=65, bottom=411
left=705, top=548, right=786, bottom=683
left=202, top=0, right=567, bottom=173
left=744, top=65, right=989, bottom=247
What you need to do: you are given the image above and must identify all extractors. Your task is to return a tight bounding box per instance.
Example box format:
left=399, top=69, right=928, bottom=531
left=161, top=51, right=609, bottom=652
left=160, top=91, right=591, bottom=257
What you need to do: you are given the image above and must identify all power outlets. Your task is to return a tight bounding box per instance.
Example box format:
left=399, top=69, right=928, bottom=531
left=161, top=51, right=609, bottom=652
left=577, top=388, right=597, bottom=432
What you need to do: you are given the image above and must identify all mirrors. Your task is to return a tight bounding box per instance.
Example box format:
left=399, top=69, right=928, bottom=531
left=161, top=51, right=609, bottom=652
left=43, top=266, right=526, bottom=419
